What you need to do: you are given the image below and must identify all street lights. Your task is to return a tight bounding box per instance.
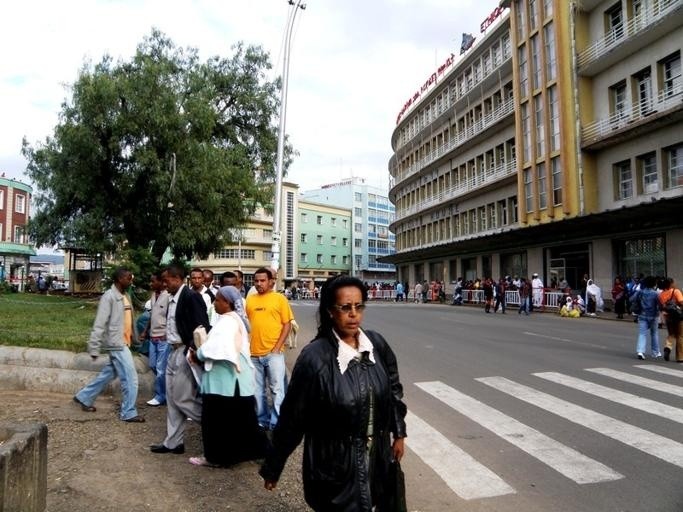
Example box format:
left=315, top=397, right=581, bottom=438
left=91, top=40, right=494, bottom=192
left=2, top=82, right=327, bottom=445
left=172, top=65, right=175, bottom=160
left=269, top=0, right=305, bottom=293
left=20, top=265, right=24, bottom=292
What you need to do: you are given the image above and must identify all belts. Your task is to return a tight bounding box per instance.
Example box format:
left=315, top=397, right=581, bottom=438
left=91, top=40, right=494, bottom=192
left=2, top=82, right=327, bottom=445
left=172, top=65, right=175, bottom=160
left=169, top=343, right=183, bottom=352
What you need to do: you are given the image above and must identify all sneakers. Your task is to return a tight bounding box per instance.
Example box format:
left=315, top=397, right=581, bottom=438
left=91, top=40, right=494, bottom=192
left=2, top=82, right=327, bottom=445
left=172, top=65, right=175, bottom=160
left=146, top=398, right=160, bottom=406
left=663, top=348, right=670, bottom=362
left=188, top=456, right=219, bottom=467
left=637, top=353, right=645, bottom=360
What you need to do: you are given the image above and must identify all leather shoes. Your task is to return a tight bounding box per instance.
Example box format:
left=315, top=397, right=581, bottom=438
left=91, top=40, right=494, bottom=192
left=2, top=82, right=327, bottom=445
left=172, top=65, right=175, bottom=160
left=73, top=396, right=95, bottom=412
left=127, top=416, right=144, bottom=423
left=150, top=444, right=184, bottom=455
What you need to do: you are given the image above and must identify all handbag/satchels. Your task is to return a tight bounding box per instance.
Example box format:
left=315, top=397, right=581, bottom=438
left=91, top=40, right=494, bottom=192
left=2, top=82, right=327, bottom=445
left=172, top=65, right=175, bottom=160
left=665, top=288, right=682, bottom=319
left=374, top=461, right=407, bottom=511
left=629, top=292, right=642, bottom=314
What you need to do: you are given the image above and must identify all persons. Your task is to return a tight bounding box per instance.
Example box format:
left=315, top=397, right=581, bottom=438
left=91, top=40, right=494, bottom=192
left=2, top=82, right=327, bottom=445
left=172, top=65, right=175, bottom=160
left=629, top=276, right=661, bottom=360
left=364, top=281, right=405, bottom=304
left=658, top=277, right=683, bottom=362
left=285, top=286, right=320, bottom=300
left=258, top=273, right=407, bottom=512
left=452, top=273, right=544, bottom=315
left=550, top=273, right=604, bottom=317
left=137, top=263, right=299, bottom=468
left=73, top=268, right=145, bottom=422
left=27, top=275, right=61, bottom=292
left=612, top=274, right=665, bottom=323
left=414, top=279, right=445, bottom=304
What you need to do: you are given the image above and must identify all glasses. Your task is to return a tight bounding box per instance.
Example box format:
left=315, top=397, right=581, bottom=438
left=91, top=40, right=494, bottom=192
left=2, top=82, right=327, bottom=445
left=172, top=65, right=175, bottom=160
left=333, top=303, right=365, bottom=313
